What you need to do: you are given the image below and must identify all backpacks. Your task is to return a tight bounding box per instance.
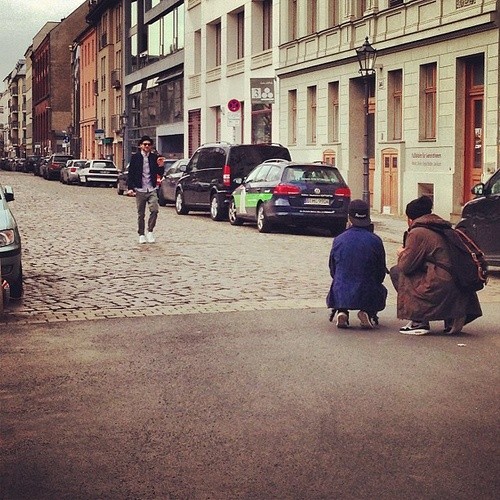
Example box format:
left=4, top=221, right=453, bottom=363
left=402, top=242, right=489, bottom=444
left=403, top=223, right=489, bottom=293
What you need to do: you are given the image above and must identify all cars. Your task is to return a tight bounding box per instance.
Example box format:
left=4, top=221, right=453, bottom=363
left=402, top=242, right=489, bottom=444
left=36, top=157, right=49, bottom=176
left=116, top=158, right=191, bottom=207
left=60, top=160, right=88, bottom=185
left=0, top=156, right=23, bottom=172
left=77, top=160, right=119, bottom=188
left=455, top=168, right=500, bottom=267
left=0, top=183, right=24, bottom=297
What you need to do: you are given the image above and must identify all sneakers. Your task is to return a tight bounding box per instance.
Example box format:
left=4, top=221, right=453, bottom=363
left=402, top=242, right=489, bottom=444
left=442, top=317, right=466, bottom=335
left=400, top=320, right=430, bottom=336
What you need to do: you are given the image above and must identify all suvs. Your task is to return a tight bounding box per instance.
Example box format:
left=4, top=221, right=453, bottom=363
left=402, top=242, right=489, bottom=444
left=229, top=159, right=351, bottom=238
left=44, top=154, right=74, bottom=180
left=23, top=156, right=39, bottom=173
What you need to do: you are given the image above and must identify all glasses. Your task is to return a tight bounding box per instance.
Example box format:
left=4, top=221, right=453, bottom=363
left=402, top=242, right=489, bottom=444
left=143, top=143, right=152, bottom=146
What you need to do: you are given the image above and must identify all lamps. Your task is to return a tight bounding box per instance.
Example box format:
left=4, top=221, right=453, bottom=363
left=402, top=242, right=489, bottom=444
left=68, top=44, right=75, bottom=52
left=111, top=80, right=120, bottom=89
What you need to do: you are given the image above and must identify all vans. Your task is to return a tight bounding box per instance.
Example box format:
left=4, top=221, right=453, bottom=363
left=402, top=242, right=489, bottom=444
left=175, top=141, right=293, bottom=221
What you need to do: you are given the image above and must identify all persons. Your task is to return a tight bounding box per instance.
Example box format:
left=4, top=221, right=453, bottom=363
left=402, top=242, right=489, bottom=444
left=390, top=195, right=482, bottom=336
left=326, top=199, right=388, bottom=329
left=127, top=135, right=165, bottom=244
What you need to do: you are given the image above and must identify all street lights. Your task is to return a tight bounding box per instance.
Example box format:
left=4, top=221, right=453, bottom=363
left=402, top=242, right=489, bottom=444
left=355, top=36, right=378, bottom=214
left=67, top=122, right=75, bottom=156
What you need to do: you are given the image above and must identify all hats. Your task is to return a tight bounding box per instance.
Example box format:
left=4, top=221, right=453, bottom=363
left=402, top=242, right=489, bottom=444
left=138, top=135, right=154, bottom=147
left=406, top=195, right=433, bottom=220
left=348, top=199, right=371, bottom=227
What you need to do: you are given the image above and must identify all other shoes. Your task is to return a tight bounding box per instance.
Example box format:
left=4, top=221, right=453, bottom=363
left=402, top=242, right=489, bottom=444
left=357, top=308, right=379, bottom=328
left=336, top=310, right=350, bottom=328
left=146, top=232, right=156, bottom=243
left=139, top=235, right=146, bottom=244
